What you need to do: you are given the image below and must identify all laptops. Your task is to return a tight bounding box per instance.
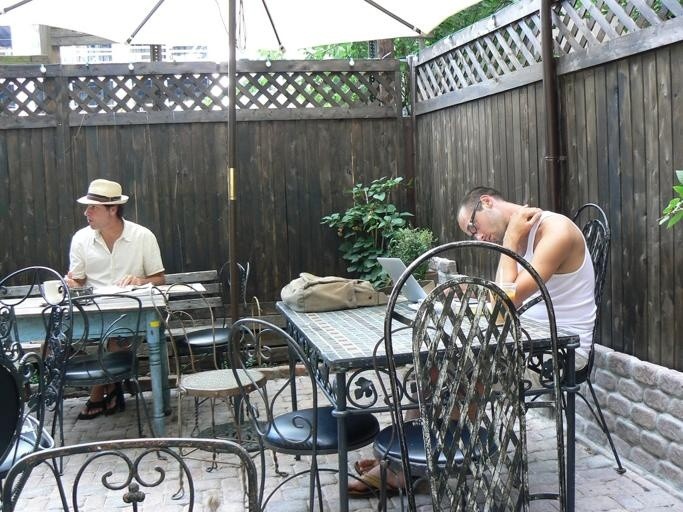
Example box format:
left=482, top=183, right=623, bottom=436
left=376, top=258, right=488, bottom=304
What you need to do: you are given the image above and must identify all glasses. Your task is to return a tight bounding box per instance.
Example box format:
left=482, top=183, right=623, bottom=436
left=466, top=200, right=481, bottom=240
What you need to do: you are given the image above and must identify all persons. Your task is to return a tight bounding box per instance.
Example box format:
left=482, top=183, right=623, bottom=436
left=61, top=177, right=166, bottom=423
left=346, top=185, right=598, bottom=499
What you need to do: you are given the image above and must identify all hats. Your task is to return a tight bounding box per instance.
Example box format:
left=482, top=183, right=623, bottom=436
left=74, top=177, right=130, bottom=206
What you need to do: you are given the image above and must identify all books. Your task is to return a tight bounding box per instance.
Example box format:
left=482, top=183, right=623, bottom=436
left=91, top=280, right=154, bottom=295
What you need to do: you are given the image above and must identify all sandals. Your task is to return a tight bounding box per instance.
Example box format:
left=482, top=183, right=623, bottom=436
left=78, top=388, right=104, bottom=420
left=104, top=387, right=125, bottom=416
left=345, top=460, right=410, bottom=499
left=354, top=458, right=436, bottom=495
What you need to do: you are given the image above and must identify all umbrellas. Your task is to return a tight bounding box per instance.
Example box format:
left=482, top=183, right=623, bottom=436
left=0, top=0, right=483, bottom=454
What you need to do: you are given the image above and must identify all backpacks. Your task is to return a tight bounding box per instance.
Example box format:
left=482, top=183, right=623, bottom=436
left=280, top=272, right=390, bottom=314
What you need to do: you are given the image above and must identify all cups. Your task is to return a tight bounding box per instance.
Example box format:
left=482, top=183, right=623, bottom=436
left=488, top=282, right=518, bottom=326
left=70, top=271, right=88, bottom=288
left=43, top=279, right=63, bottom=304
